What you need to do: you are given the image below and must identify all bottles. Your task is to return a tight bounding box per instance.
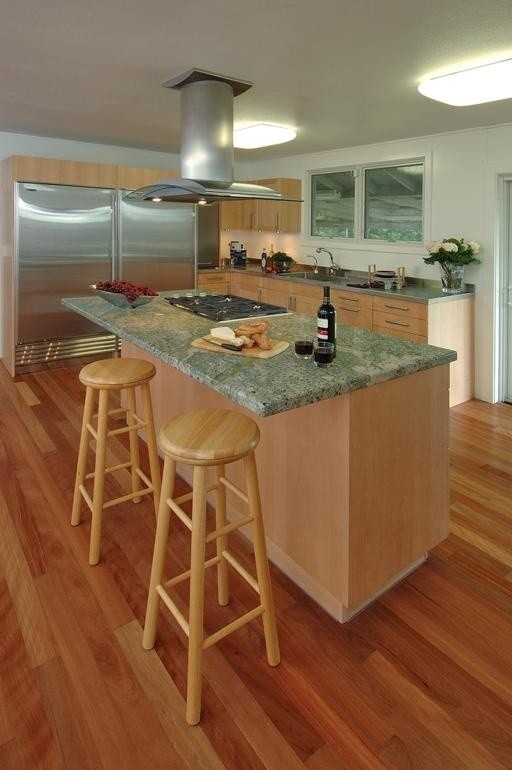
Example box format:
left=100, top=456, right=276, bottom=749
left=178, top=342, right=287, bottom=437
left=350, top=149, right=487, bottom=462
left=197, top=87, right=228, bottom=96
left=269, top=243, right=275, bottom=258
left=260, top=248, right=268, bottom=271
left=315, top=285, right=338, bottom=359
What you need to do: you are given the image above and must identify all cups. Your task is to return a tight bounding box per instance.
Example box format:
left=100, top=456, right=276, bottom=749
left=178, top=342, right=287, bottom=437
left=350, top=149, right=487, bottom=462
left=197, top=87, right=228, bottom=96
left=313, top=341, right=337, bottom=370
left=383, top=281, right=404, bottom=290
left=398, top=266, right=407, bottom=286
left=294, top=336, right=314, bottom=361
left=368, top=264, right=377, bottom=283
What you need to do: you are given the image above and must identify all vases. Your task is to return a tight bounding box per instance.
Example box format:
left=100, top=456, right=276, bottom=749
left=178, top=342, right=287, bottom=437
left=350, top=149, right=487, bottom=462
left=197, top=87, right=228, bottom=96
left=438, top=263, right=469, bottom=292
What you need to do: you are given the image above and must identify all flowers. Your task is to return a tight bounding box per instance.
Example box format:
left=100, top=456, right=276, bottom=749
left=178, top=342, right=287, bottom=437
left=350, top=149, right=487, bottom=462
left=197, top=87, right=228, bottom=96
left=422, top=236, right=484, bottom=290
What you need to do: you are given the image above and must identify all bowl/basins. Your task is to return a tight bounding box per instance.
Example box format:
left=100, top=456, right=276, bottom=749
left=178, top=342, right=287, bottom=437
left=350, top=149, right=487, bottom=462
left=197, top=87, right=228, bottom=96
left=89, top=283, right=158, bottom=310
left=273, top=261, right=296, bottom=272
left=372, top=270, right=398, bottom=283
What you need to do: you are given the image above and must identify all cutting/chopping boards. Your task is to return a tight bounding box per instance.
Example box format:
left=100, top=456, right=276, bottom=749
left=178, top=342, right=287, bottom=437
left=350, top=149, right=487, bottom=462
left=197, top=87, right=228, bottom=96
left=189, top=333, right=292, bottom=359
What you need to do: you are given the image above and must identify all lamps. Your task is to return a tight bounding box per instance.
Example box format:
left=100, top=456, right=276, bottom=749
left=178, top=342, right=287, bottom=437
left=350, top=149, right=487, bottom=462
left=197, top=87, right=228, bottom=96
left=233, top=118, right=301, bottom=152
left=413, top=47, right=512, bottom=110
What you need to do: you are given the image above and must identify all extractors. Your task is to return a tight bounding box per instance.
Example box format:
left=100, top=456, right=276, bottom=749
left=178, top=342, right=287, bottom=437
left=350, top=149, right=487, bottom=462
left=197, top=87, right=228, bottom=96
left=117, top=68, right=304, bottom=207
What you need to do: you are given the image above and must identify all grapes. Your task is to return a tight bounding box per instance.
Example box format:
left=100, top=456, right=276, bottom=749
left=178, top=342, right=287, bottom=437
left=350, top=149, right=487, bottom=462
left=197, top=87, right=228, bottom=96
left=96, top=280, right=158, bottom=303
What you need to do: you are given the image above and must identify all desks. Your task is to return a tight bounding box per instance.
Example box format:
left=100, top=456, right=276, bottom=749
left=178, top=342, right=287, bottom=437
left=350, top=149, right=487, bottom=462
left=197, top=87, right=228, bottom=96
left=52, top=287, right=460, bottom=627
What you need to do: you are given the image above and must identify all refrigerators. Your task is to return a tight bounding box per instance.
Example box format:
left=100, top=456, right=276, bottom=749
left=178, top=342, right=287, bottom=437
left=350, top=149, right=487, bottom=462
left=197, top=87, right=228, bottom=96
left=12, top=181, right=197, bottom=375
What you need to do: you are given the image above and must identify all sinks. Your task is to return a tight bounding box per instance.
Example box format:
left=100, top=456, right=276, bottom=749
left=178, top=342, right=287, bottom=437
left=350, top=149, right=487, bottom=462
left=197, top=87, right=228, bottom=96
left=275, top=271, right=351, bottom=281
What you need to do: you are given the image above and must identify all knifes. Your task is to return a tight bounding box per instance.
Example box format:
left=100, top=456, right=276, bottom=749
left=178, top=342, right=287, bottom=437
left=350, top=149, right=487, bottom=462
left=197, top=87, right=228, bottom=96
left=199, top=336, right=240, bottom=351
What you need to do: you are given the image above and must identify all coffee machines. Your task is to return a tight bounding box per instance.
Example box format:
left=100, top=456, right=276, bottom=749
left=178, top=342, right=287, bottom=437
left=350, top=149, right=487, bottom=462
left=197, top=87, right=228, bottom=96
left=228, top=241, right=247, bottom=269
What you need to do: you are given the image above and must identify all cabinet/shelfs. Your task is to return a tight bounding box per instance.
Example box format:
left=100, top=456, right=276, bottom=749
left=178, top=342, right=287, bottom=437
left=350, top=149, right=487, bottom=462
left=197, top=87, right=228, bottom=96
left=328, top=283, right=373, bottom=330
left=229, top=270, right=260, bottom=303
left=261, top=275, right=324, bottom=315
left=196, top=271, right=229, bottom=301
left=219, top=178, right=302, bottom=233
left=374, top=292, right=471, bottom=408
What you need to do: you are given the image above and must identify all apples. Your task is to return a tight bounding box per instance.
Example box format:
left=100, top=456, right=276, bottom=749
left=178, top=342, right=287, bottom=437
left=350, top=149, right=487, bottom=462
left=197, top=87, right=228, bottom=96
left=265, top=266, right=273, bottom=272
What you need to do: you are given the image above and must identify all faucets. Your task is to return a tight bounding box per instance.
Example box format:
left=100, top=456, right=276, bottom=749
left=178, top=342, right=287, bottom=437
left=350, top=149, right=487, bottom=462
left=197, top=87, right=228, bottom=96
left=307, top=255, right=320, bottom=273
left=315, top=247, right=335, bottom=273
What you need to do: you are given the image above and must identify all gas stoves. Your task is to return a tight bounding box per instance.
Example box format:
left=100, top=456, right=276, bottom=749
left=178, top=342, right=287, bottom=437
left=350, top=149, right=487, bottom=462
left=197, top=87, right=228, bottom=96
left=164, top=295, right=289, bottom=322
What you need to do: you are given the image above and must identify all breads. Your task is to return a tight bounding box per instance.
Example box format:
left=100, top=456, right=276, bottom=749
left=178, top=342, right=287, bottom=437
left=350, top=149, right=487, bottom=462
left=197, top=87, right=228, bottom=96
left=237, top=322, right=267, bottom=334
left=239, top=336, right=255, bottom=348
left=252, top=333, right=273, bottom=350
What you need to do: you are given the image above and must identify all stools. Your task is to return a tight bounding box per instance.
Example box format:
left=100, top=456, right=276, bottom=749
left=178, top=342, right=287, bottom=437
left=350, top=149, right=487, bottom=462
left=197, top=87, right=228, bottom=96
left=70, top=357, right=161, bottom=568
left=139, top=404, right=283, bottom=731
